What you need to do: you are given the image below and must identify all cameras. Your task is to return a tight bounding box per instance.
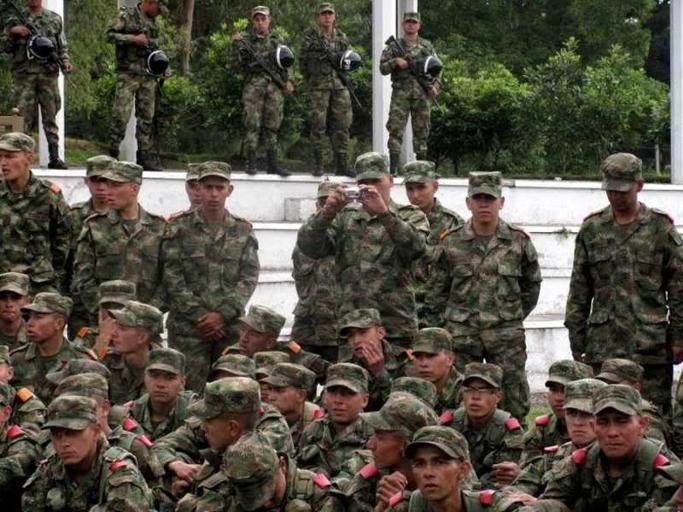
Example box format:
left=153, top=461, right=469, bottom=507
left=342, top=187, right=362, bottom=200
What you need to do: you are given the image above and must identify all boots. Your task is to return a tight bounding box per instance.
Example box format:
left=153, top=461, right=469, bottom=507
left=312, top=145, right=325, bottom=176
left=246, top=150, right=257, bottom=174
left=417, top=152, right=440, bottom=178
left=48, top=144, right=67, bottom=169
left=109, top=148, right=120, bottom=161
left=334, top=153, right=357, bottom=178
left=390, top=152, right=402, bottom=177
left=137, top=148, right=162, bottom=171
left=267, top=149, right=290, bottom=176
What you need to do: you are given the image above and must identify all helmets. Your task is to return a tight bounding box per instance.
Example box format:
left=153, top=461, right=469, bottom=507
left=28, top=34, right=55, bottom=59
left=146, top=50, right=169, bottom=76
left=273, top=43, right=295, bottom=70
left=421, top=56, right=443, bottom=78
left=339, top=49, right=362, bottom=70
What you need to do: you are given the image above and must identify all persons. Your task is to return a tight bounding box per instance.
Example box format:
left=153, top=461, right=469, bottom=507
left=391, top=377, right=438, bottom=408
left=1, top=0, right=73, bottom=170
left=412, top=327, right=465, bottom=416
left=99, top=300, right=207, bottom=408
left=297, top=152, right=430, bottom=349
left=149, top=355, right=297, bottom=496
left=151, top=377, right=298, bottom=499
left=72, top=161, right=168, bottom=329
left=440, top=362, right=526, bottom=480
left=44, top=358, right=152, bottom=448
left=253, top=351, right=290, bottom=380
left=1, top=272, right=33, bottom=358
left=379, top=12, right=443, bottom=177
left=336, top=308, right=415, bottom=413
left=0, top=384, right=42, bottom=512
left=1, top=345, right=47, bottom=433
left=232, top=6, right=295, bottom=178
left=222, top=304, right=334, bottom=357
left=598, top=357, right=673, bottom=451
left=125, top=348, right=203, bottom=442
left=297, top=363, right=375, bottom=493
left=168, top=163, right=203, bottom=222
left=492, top=377, right=608, bottom=512
left=346, top=390, right=479, bottom=512
left=162, top=161, right=260, bottom=399
left=103, top=0, right=169, bottom=171
left=259, top=363, right=325, bottom=451
left=490, top=360, right=596, bottom=483
left=564, top=153, right=683, bottom=451
left=384, top=425, right=525, bottom=512
left=72, top=279, right=163, bottom=352
left=654, top=463, right=683, bottom=512
left=61, top=155, right=117, bottom=342
left=9, top=292, right=99, bottom=407
left=40, top=372, right=165, bottom=487
left=176, top=430, right=352, bottom=512
left=516, top=384, right=683, bottom=512
left=1, top=131, right=73, bottom=299
left=20, top=396, right=153, bottom=512
left=425, top=171, right=543, bottom=433
left=300, top=3, right=362, bottom=178
left=400, top=160, right=465, bottom=327
left=291, top=182, right=349, bottom=364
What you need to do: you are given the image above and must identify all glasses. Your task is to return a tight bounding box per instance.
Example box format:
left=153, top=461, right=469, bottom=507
left=462, top=384, right=493, bottom=394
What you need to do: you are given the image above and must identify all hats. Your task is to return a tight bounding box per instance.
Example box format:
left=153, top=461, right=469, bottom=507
left=20, top=292, right=74, bottom=321
left=411, top=326, right=455, bottom=355
left=252, top=351, right=289, bottom=377
left=601, top=151, right=642, bottom=192
left=0, top=272, right=30, bottom=296
left=57, top=372, right=109, bottom=401
left=197, top=160, right=232, bottom=182
left=258, top=362, right=316, bottom=392
left=383, top=376, right=437, bottom=409
left=107, top=300, right=164, bottom=335
left=185, top=377, right=262, bottom=421
left=41, top=395, right=98, bottom=432
left=211, top=353, right=256, bottom=378
left=468, top=171, right=503, bottom=199
left=338, top=308, right=382, bottom=337
left=0, top=344, right=11, bottom=364
left=316, top=181, right=338, bottom=198
left=236, top=304, right=286, bottom=335
left=358, top=393, right=440, bottom=438
left=157, top=0, right=169, bottom=13
left=147, top=348, right=185, bottom=375
left=45, top=358, right=112, bottom=385
left=185, top=163, right=201, bottom=181
left=400, top=160, right=436, bottom=186
left=0, top=132, right=35, bottom=152
left=96, top=160, right=143, bottom=185
left=222, top=429, right=278, bottom=512
left=97, top=279, right=135, bottom=306
left=318, top=2, right=334, bottom=14
left=325, top=362, right=368, bottom=393
left=592, top=383, right=642, bottom=417
left=563, top=378, right=607, bottom=414
left=354, top=151, right=389, bottom=182
left=404, top=12, right=421, bottom=23
left=404, top=424, right=469, bottom=460
left=86, top=155, right=117, bottom=178
left=462, top=362, right=503, bottom=390
left=0, top=383, right=9, bottom=406
left=594, top=358, right=644, bottom=382
left=544, top=359, right=593, bottom=388
left=251, top=5, right=270, bottom=17
